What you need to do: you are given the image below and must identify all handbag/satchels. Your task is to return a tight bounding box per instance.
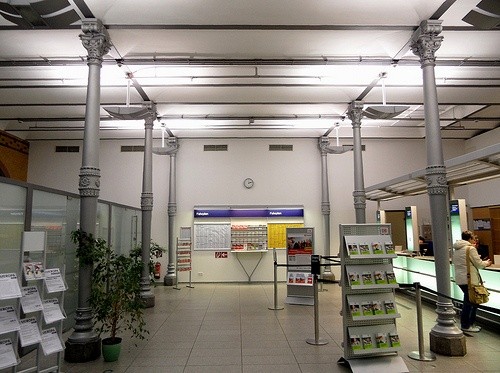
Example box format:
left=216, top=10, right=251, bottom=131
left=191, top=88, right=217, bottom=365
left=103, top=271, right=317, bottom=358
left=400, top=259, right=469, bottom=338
left=467, top=284, right=489, bottom=304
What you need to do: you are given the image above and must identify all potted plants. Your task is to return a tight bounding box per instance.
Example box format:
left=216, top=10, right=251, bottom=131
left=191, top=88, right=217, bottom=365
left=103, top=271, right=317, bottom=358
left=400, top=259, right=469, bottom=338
left=68, top=228, right=149, bottom=364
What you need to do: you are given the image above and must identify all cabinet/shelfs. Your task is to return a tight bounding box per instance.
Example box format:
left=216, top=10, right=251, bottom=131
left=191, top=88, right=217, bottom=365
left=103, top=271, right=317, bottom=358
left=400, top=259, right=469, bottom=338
left=338, top=223, right=407, bottom=373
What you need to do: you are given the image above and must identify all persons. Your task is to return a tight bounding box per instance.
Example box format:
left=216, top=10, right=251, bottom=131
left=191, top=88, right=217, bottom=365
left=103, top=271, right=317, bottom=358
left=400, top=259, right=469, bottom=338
left=418, top=236, right=425, bottom=244
left=288, top=237, right=312, bottom=249
left=474, top=235, right=484, bottom=258
left=452, top=231, right=492, bottom=332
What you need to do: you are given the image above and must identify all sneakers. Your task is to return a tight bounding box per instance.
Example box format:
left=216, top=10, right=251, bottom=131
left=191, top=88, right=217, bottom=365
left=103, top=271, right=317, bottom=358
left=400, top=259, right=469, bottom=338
left=461, top=324, right=482, bottom=333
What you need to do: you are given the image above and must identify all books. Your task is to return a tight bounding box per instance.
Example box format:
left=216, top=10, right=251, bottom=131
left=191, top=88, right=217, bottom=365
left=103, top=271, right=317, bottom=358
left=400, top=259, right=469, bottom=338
left=348, top=241, right=395, bottom=255
left=348, top=270, right=397, bottom=285
left=350, top=300, right=396, bottom=316
left=350, top=331, right=401, bottom=350
